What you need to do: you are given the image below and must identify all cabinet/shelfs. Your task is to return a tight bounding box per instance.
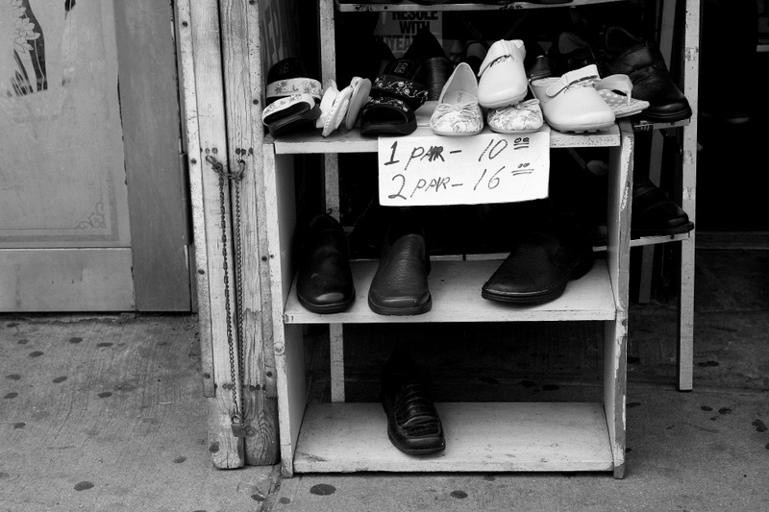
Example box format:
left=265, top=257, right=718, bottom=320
left=317, top=0, right=703, bottom=404
left=260, top=121, right=639, bottom=479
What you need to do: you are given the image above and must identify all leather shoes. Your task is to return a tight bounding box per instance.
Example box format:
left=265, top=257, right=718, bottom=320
left=382, top=378, right=446, bottom=455
left=632, top=165, right=694, bottom=237
left=367, top=234, right=432, bottom=316
left=481, top=226, right=595, bottom=306
left=294, top=210, right=356, bottom=315
left=431, top=19, right=691, bottom=135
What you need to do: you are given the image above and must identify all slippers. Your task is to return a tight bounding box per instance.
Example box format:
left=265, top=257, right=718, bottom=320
left=260, top=41, right=426, bottom=142
